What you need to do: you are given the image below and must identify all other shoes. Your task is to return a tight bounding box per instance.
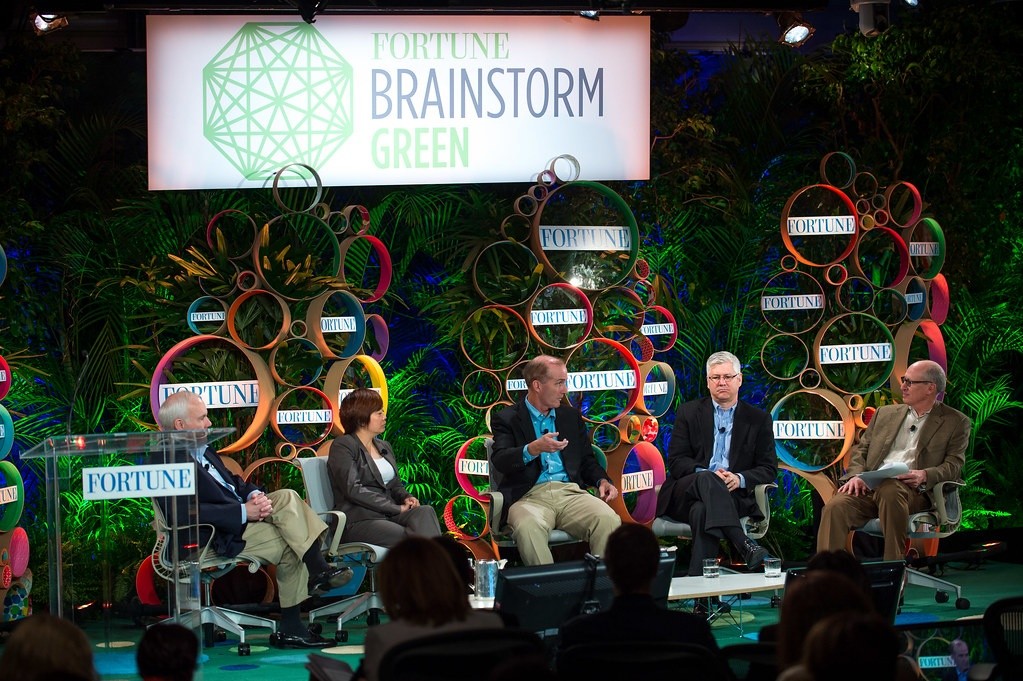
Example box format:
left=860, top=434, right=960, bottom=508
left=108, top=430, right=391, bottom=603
left=737, top=539, right=769, bottom=571
left=694, top=596, right=731, bottom=613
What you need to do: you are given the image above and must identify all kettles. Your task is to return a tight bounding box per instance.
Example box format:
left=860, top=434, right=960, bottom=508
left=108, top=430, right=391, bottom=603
left=469, top=559, right=508, bottom=602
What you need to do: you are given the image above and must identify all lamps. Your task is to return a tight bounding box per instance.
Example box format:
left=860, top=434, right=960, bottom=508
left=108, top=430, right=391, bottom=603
left=777, top=13, right=815, bottom=51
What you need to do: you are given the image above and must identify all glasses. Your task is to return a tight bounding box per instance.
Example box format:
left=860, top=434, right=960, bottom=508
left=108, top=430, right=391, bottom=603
left=900, top=376, right=933, bottom=388
left=708, top=373, right=739, bottom=382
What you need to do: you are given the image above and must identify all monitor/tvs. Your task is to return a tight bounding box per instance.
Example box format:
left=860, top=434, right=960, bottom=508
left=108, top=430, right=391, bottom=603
left=780, top=560, right=907, bottom=627
left=494, top=550, right=676, bottom=648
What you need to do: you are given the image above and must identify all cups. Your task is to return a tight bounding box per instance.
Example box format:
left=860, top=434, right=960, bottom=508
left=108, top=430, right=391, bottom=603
left=763, top=558, right=781, bottom=577
left=701, top=558, right=720, bottom=579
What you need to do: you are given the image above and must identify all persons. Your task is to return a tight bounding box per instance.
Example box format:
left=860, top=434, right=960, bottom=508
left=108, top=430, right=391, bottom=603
left=943, top=638, right=975, bottom=681
left=488, top=354, right=623, bottom=566
left=773, top=552, right=928, bottom=681
left=135, top=624, right=200, bottom=681
left=304, top=653, right=353, bottom=681
left=552, top=521, right=740, bottom=681
left=0, top=615, right=102, bottom=681
left=816, top=360, right=973, bottom=608
left=655, top=352, right=778, bottom=614
left=361, top=531, right=504, bottom=681
left=326, top=389, right=442, bottom=549
left=142, top=393, right=355, bottom=648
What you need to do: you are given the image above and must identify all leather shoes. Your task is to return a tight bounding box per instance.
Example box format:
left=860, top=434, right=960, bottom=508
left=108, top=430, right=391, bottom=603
left=307, top=566, right=353, bottom=596
left=276, top=629, right=337, bottom=648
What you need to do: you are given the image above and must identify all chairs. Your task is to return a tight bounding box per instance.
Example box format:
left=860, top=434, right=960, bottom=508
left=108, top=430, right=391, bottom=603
left=478, top=438, right=608, bottom=560
left=983, top=597, right=1023, bottom=681
left=719, top=642, right=778, bottom=681
left=554, top=640, right=722, bottom=681
left=297, top=456, right=392, bottom=641
left=376, top=626, right=551, bottom=681
left=149, top=496, right=286, bottom=654
left=651, top=481, right=780, bottom=610
left=839, top=468, right=970, bottom=614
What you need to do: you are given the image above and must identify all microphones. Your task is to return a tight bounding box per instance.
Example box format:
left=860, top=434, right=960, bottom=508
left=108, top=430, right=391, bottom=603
left=544, top=428, right=549, bottom=434
left=382, top=448, right=388, bottom=454
left=204, top=463, right=210, bottom=470
left=910, top=425, right=915, bottom=431
left=720, top=426, right=725, bottom=433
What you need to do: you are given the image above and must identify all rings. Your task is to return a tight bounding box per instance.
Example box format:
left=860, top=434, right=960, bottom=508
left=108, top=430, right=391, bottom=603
left=733, top=480, right=735, bottom=484
